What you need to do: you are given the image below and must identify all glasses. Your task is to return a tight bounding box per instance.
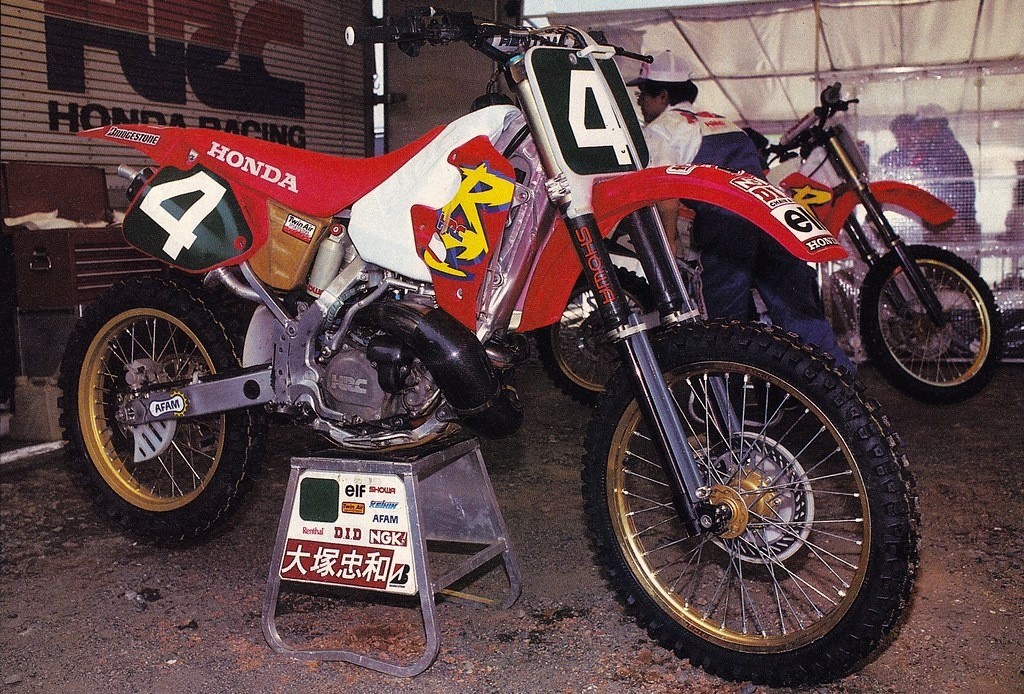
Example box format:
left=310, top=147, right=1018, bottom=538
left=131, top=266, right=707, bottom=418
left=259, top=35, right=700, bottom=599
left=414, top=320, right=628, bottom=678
left=634, top=90, right=644, bottom=100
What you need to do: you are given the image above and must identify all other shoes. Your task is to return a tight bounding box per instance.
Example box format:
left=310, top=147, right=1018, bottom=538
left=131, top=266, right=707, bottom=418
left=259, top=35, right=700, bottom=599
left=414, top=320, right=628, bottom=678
left=779, top=397, right=801, bottom=412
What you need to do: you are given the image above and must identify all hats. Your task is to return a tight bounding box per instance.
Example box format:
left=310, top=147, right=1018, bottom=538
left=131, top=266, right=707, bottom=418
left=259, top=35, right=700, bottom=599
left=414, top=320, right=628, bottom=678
left=911, top=103, right=949, bottom=124
left=625, top=49, right=693, bottom=87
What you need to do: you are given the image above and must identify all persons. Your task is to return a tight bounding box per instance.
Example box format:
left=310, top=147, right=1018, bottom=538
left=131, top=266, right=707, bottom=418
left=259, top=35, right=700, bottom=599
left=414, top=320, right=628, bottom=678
left=877, top=103, right=983, bottom=289
left=625, top=50, right=859, bottom=429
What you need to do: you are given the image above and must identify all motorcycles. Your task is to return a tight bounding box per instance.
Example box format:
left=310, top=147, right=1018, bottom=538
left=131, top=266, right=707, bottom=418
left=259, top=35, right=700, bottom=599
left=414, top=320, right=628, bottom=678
left=532, top=81, right=1008, bottom=403
left=56, top=5, right=922, bottom=689
